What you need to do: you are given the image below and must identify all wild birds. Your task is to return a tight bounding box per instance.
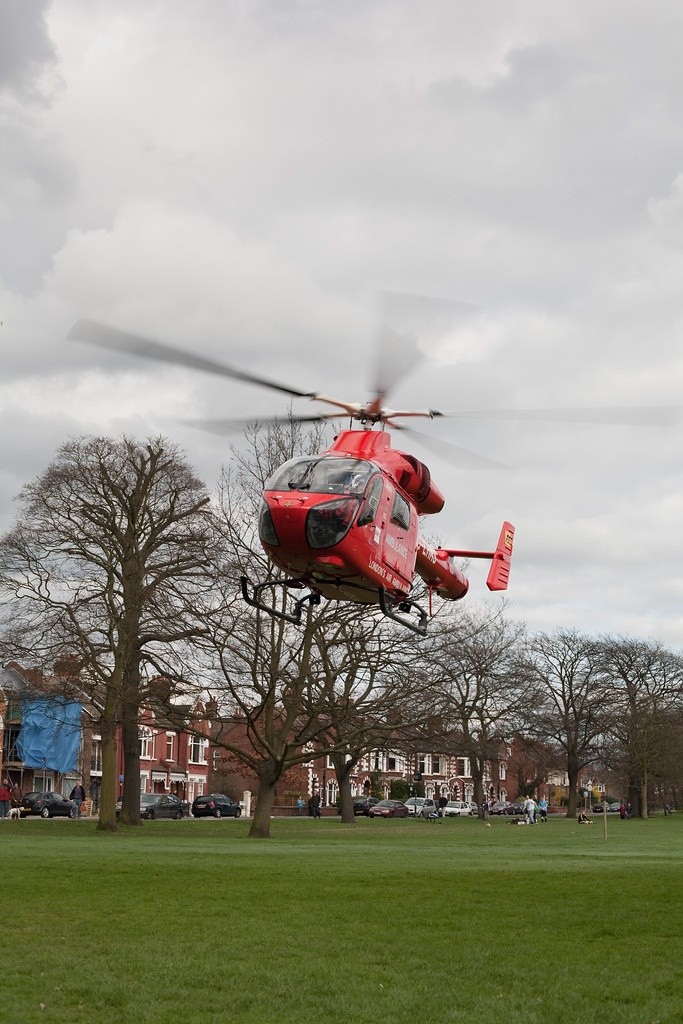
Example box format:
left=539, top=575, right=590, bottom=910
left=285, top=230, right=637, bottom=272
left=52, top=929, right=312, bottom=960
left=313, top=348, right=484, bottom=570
left=486, top=823, right=491, bottom=828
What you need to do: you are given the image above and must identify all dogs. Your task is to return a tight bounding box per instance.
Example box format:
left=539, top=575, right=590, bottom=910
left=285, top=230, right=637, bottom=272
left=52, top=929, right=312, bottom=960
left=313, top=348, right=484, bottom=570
left=505, top=818, right=530, bottom=826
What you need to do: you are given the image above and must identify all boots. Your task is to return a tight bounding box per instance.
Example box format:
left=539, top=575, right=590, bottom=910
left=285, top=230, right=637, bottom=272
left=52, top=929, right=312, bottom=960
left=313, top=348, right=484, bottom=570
left=545, top=818, right=548, bottom=823
left=541, top=819, right=543, bottom=823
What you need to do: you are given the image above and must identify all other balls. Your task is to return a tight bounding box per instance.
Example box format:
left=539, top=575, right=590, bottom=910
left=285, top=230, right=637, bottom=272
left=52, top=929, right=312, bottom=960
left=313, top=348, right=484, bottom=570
left=486, top=823, right=490, bottom=828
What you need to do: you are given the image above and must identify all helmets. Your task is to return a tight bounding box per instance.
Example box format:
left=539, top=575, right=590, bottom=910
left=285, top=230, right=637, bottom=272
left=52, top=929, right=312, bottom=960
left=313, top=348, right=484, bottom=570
left=351, top=475, right=365, bottom=494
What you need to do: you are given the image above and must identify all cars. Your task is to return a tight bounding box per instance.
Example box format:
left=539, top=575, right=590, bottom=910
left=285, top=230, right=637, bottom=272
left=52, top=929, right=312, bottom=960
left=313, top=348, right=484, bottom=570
left=488, top=800, right=526, bottom=817
left=593, top=803, right=604, bottom=813
left=115, top=795, right=125, bottom=817
left=139, top=792, right=191, bottom=821
left=21, top=790, right=78, bottom=819
left=463, top=800, right=484, bottom=815
left=606, top=802, right=621, bottom=813
left=353, top=795, right=381, bottom=817
left=369, top=799, right=409, bottom=819
left=405, top=796, right=436, bottom=819
left=438, top=801, right=475, bottom=818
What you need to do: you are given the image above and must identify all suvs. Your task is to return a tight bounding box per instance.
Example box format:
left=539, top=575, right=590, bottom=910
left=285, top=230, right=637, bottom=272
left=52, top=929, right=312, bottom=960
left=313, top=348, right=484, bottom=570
left=193, top=792, right=243, bottom=819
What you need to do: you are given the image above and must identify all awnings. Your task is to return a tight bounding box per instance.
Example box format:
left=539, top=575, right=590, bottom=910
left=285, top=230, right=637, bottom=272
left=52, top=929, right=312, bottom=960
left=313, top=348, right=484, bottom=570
left=152, top=772, right=186, bottom=783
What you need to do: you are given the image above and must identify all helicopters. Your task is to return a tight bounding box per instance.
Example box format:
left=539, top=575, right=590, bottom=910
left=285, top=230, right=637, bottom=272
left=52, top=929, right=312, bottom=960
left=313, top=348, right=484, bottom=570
left=72, top=293, right=682, bottom=637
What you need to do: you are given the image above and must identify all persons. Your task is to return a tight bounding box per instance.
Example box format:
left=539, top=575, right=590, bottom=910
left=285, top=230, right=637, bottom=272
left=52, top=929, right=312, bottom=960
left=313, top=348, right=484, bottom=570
left=69, top=780, right=87, bottom=820
left=522, top=794, right=549, bottom=824
left=439, top=794, right=448, bottom=819
left=578, top=811, right=594, bottom=824
left=0, top=778, right=24, bottom=820
left=298, top=789, right=321, bottom=819
left=480, top=801, right=491, bottom=822
left=619, top=803, right=632, bottom=819
